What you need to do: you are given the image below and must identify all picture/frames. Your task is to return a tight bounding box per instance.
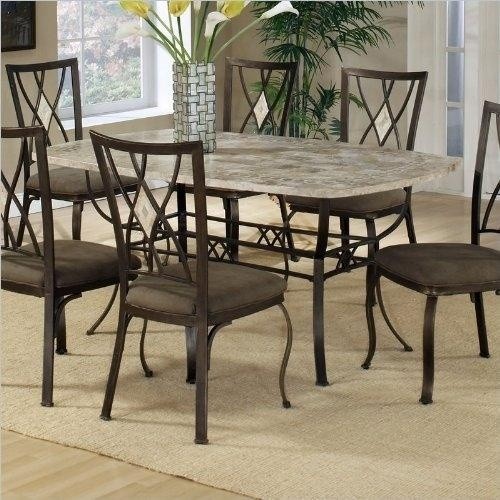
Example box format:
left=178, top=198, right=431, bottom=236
left=1, top=1, right=36, bottom=52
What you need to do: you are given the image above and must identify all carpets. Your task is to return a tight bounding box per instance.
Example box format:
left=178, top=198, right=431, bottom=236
left=1, top=184, right=500, bottom=500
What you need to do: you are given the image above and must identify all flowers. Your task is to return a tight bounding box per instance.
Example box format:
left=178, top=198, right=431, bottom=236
left=121, top=1, right=299, bottom=60
